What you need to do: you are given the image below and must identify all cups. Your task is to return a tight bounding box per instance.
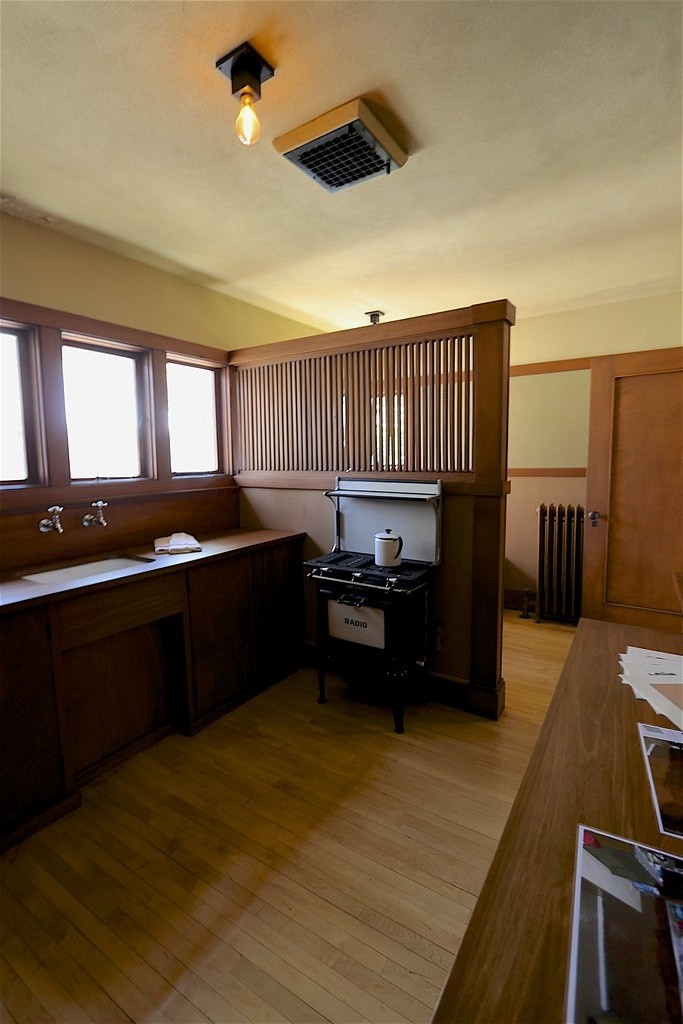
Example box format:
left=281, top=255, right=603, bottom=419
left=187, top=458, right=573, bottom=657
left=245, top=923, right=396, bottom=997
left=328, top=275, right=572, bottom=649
left=375, top=529, right=403, bottom=566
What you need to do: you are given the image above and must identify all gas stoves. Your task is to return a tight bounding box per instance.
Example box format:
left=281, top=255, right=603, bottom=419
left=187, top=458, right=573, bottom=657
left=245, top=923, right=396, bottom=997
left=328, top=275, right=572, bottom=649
left=304, top=548, right=434, bottom=595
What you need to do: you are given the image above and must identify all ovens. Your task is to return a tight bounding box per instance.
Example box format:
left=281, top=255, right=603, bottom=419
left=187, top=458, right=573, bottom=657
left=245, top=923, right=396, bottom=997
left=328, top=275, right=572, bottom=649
left=315, top=581, right=428, bottom=689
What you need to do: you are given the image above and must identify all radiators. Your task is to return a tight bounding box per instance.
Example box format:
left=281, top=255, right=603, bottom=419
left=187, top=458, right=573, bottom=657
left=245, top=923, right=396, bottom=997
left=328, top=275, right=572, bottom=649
left=519, top=502, right=585, bottom=625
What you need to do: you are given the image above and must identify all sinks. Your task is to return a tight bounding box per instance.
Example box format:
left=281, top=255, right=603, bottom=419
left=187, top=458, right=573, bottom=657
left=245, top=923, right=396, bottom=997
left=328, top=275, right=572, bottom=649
left=16, top=552, right=156, bottom=586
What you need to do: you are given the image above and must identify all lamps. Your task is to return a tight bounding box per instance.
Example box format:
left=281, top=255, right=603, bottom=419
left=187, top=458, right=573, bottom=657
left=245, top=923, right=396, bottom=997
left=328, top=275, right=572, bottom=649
left=216, top=42, right=276, bottom=146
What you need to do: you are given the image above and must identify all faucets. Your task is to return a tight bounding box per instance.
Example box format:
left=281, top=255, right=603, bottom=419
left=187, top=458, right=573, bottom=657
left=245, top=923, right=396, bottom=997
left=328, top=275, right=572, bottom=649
left=81, top=500, right=109, bottom=528
left=38, top=505, right=64, bottom=535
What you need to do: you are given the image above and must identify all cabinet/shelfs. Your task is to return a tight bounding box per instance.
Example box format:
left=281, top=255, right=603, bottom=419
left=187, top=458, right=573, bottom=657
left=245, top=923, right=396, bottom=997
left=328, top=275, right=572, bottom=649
left=1, top=537, right=309, bottom=853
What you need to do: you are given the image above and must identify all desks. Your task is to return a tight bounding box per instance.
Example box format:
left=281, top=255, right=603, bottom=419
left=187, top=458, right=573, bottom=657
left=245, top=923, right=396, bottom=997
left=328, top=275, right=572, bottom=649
left=431, top=618, right=683, bottom=1024
